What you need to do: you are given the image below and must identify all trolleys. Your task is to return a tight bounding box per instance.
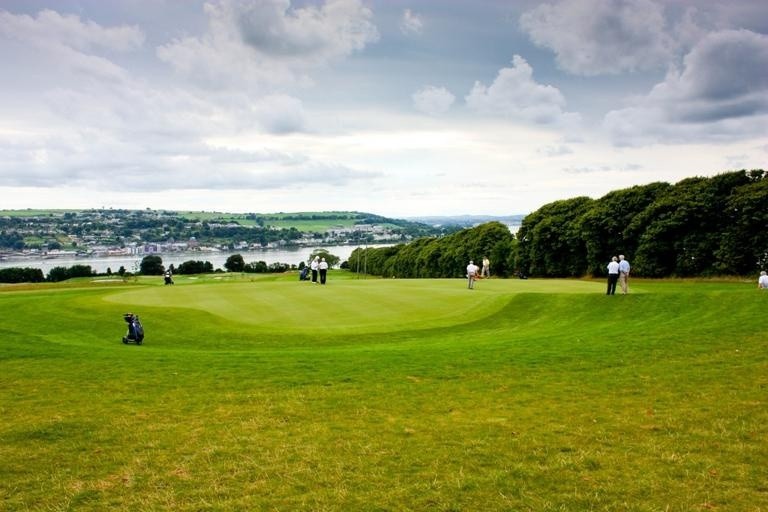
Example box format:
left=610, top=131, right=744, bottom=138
left=122, top=313, right=144, bottom=344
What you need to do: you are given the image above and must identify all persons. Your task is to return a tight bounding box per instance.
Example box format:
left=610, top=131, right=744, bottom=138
left=480, top=255, right=490, bottom=279
left=319, top=257, right=328, bottom=284
left=310, top=256, right=319, bottom=283
left=606, top=256, right=619, bottom=295
left=757, top=271, right=768, bottom=289
left=466, top=261, right=476, bottom=289
left=618, top=254, right=631, bottom=294
left=473, top=265, right=480, bottom=277
left=164, top=269, right=173, bottom=285
